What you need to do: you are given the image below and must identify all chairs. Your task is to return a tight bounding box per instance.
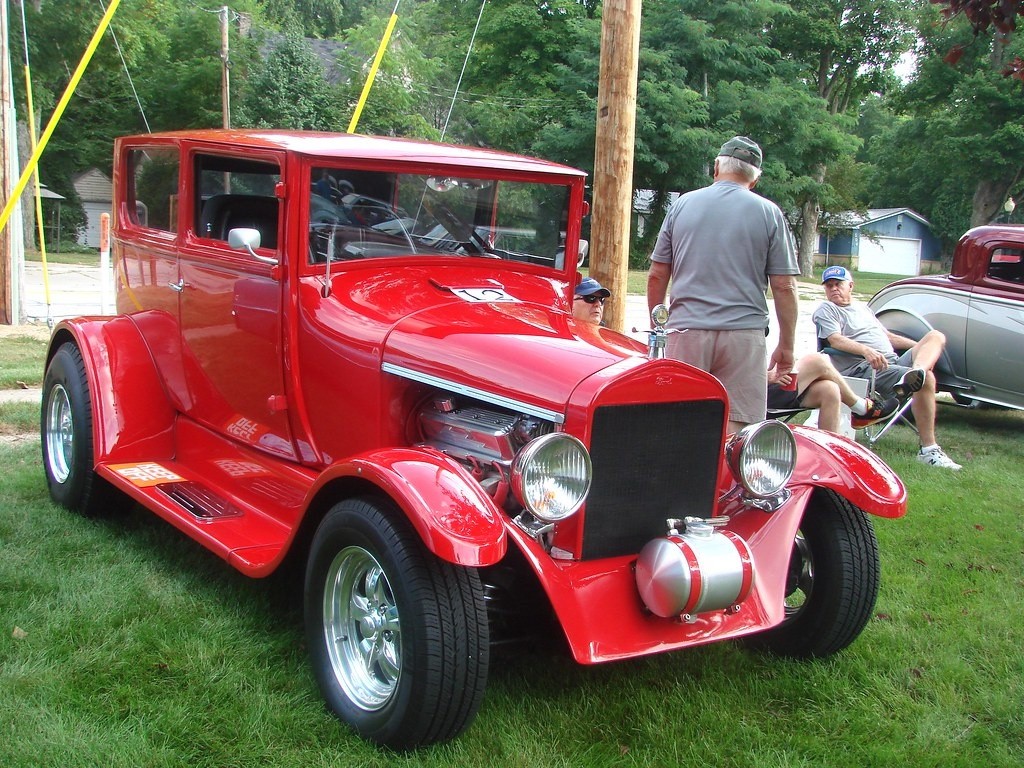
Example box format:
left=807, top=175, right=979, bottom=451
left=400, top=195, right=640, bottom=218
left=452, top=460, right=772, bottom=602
left=198, top=194, right=279, bottom=249
left=766, top=407, right=817, bottom=423
left=815, top=323, right=919, bottom=443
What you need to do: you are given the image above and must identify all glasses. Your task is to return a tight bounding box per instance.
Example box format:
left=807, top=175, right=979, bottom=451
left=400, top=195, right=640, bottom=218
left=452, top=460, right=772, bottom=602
left=572, top=294, right=606, bottom=304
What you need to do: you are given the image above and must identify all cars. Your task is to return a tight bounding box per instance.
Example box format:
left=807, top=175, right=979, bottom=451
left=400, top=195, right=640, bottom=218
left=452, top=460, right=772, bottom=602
left=42, top=131, right=909, bottom=752
left=868, top=225, right=1024, bottom=412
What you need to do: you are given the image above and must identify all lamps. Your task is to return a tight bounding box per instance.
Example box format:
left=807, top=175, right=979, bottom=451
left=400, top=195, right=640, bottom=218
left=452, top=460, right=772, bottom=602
left=897, top=224, right=902, bottom=229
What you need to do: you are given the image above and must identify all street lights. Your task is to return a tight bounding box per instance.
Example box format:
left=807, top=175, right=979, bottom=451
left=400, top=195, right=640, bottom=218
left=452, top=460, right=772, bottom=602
left=1003, top=195, right=1016, bottom=225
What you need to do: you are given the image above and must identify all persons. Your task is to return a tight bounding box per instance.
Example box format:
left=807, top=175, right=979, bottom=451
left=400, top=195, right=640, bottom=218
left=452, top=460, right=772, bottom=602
left=767, top=350, right=900, bottom=436
left=571, top=276, right=611, bottom=326
left=812, top=266, right=963, bottom=470
left=647, top=136, right=800, bottom=436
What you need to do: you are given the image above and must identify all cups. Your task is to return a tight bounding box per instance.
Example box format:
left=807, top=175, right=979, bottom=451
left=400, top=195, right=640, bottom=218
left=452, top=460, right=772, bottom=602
left=780, top=372, right=798, bottom=391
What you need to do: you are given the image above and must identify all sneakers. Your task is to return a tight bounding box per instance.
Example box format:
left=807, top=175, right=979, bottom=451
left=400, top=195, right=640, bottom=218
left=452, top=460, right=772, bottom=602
left=893, top=367, right=926, bottom=397
left=916, top=445, right=963, bottom=469
left=850, top=397, right=900, bottom=430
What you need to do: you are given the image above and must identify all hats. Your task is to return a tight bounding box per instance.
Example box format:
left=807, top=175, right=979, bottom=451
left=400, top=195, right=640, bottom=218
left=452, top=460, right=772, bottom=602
left=719, top=135, right=762, bottom=169
left=572, top=276, right=611, bottom=298
left=820, top=265, right=853, bottom=285
